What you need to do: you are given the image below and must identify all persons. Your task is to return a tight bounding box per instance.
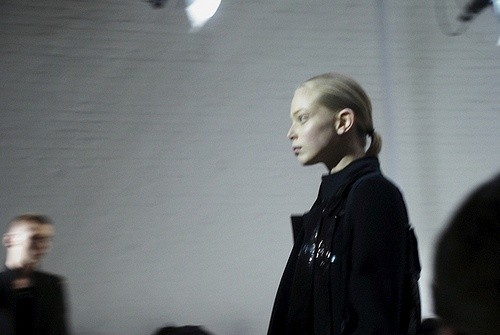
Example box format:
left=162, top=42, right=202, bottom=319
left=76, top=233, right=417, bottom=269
left=0, top=213, right=71, bottom=335
left=267, top=72, right=421, bottom=335
